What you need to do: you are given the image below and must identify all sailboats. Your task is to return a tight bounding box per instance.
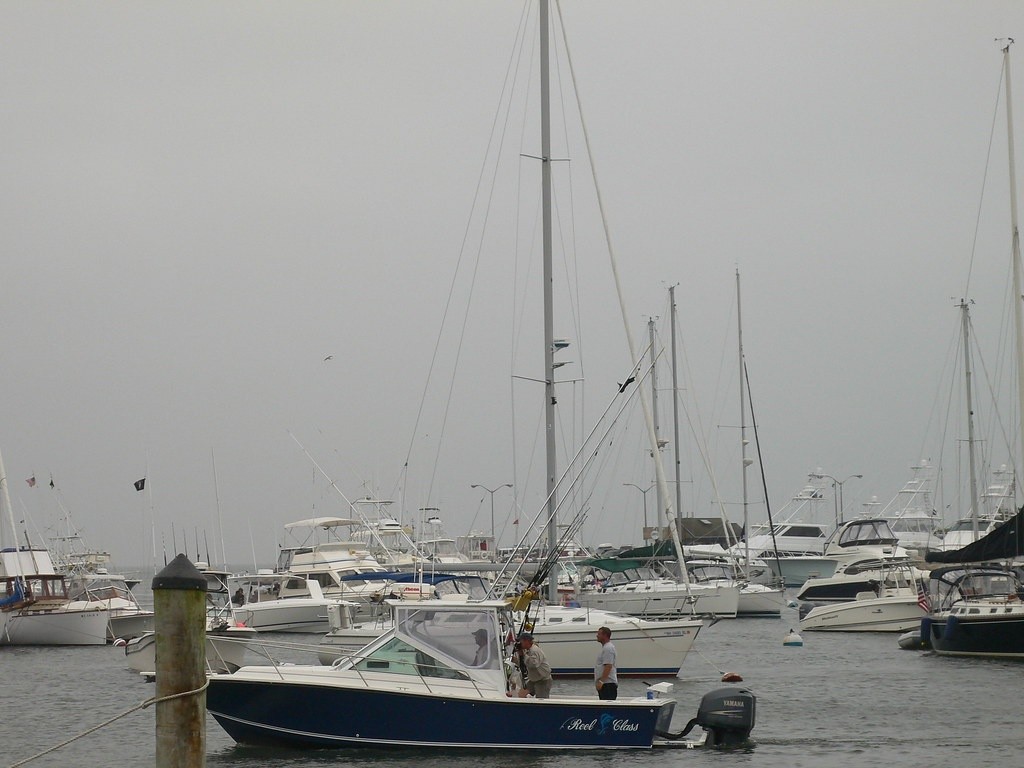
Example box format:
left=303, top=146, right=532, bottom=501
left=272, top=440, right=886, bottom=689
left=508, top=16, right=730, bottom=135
left=0, top=0, right=1024, bottom=663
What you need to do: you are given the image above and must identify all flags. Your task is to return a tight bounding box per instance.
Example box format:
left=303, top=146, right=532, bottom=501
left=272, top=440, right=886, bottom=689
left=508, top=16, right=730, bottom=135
left=25, top=477, right=36, bottom=488
left=915, top=578, right=930, bottom=613
left=133, top=479, right=145, bottom=491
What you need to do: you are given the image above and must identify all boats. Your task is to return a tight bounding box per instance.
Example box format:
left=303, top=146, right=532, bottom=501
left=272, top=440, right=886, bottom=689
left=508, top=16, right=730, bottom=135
left=137, top=347, right=756, bottom=752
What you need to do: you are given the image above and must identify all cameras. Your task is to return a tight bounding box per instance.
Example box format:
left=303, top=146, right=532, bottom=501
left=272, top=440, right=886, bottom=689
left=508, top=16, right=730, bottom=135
left=515, top=641, right=522, bottom=650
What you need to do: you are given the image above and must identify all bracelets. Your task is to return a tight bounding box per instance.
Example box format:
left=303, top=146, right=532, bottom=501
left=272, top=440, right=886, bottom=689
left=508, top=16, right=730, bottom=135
left=599, top=680, right=603, bottom=684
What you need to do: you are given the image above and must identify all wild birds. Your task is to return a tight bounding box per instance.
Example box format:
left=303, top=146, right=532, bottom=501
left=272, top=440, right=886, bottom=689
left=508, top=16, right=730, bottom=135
left=324, top=356, right=333, bottom=361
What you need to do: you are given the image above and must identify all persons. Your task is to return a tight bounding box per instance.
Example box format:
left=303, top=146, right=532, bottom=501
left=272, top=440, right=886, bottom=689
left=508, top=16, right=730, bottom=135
left=231, top=581, right=269, bottom=606
left=273, top=581, right=280, bottom=596
left=511, top=633, right=553, bottom=699
left=471, top=629, right=487, bottom=666
left=594, top=626, right=619, bottom=700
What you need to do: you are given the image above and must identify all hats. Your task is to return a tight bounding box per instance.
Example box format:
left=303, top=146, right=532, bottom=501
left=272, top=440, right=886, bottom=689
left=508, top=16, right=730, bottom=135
left=517, top=633, right=534, bottom=641
left=472, top=629, right=487, bottom=637
